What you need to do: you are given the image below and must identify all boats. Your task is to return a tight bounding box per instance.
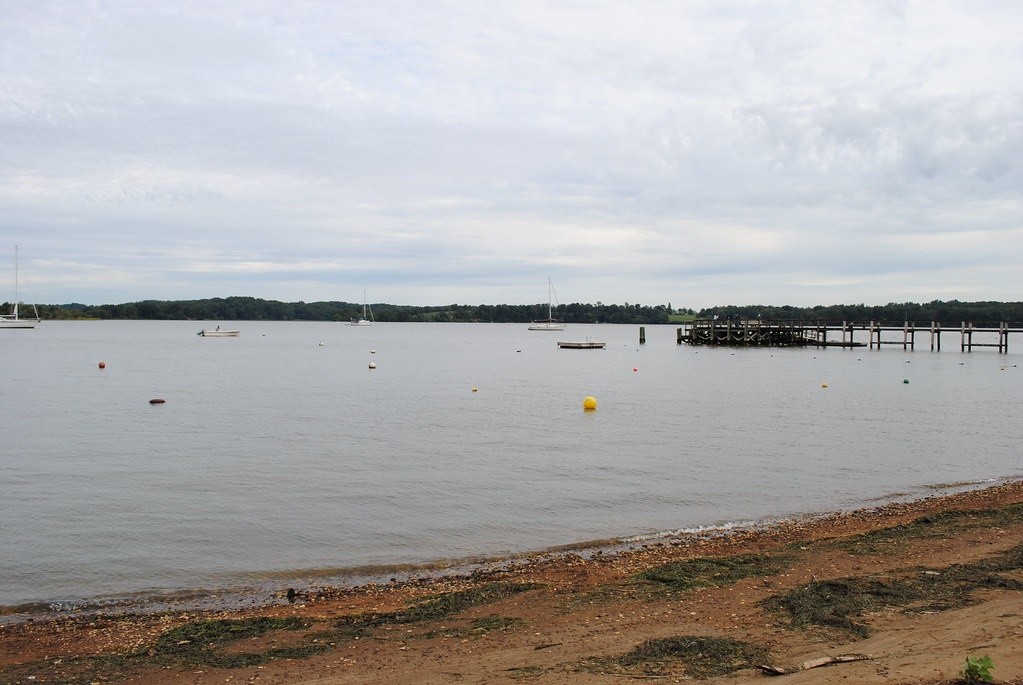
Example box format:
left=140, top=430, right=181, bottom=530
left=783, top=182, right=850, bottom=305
left=557, top=336, right=606, bottom=348
left=196, top=326, right=241, bottom=338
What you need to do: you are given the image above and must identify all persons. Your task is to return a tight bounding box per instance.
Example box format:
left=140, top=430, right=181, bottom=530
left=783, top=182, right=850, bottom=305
left=215, top=326, right=220, bottom=331
left=713, top=314, right=741, bottom=328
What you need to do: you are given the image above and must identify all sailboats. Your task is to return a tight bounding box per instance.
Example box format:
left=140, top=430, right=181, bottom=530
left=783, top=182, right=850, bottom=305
left=527, top=277, right=568, bottom=330
left=0, top=244, right=41, bottom=328
left=346, top=289, right=376, bottom=326
